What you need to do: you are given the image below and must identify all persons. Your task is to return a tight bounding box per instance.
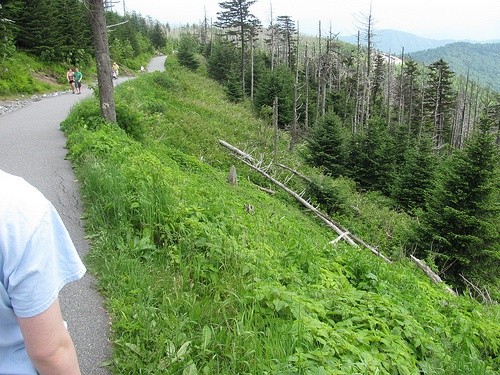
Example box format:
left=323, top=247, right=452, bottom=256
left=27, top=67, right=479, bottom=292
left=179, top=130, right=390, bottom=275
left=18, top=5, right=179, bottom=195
left=67, top=69, right=75, bottom=94
left=0, top=169, right=82, bottom=375
left=73, top=68, right=82, bottom=95
left=112, top=61, right=119, bottom=80
